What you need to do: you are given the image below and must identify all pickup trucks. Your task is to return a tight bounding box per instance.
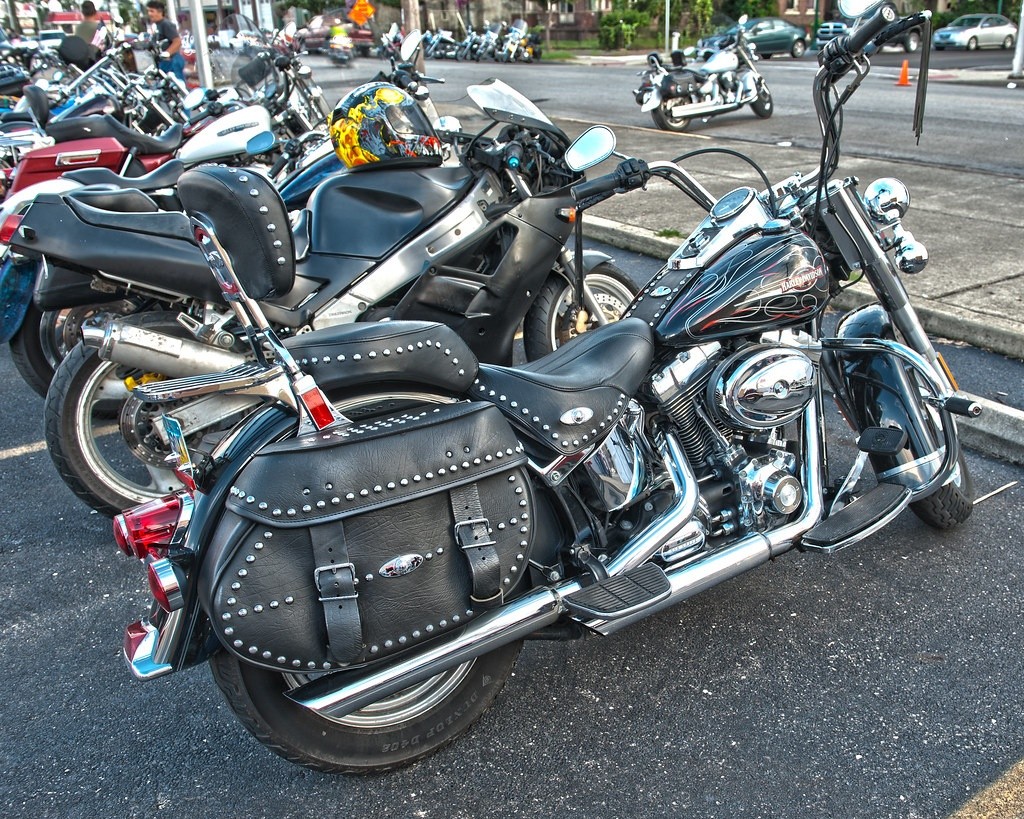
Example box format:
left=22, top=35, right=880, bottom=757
left=815, top=0, right=932, bottom=53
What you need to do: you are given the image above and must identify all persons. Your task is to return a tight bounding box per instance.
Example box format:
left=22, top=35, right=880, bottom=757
left=8, top=29, right=19, bottom=45
left=146, top=1, right=184, bottom=80
left=325, top=21, right=348, bottom=41
left=74, top=0, right=100, bottom=45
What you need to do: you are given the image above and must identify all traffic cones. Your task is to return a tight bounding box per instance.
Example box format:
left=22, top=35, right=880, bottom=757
left=895, top=59, right=912, bottom=87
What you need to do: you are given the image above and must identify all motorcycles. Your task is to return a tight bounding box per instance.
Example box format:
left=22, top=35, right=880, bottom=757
left=0, top=1, right=640, bottom=523
left=111, top=0, right=982, bottom=778
left=632, top=15, right=773, bottom=133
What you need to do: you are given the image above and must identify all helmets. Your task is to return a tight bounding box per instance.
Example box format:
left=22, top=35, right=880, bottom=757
left=327, top=81, right=444, bottom=174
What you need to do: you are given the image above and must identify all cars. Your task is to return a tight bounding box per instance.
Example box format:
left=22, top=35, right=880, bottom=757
left=34, top=29, right=68, bottom=50
left=292, top=9, right=372, bottom=59
left=932, top=12, right=1018, bottom=52
left=700, top=16, right=811, bottom=62
